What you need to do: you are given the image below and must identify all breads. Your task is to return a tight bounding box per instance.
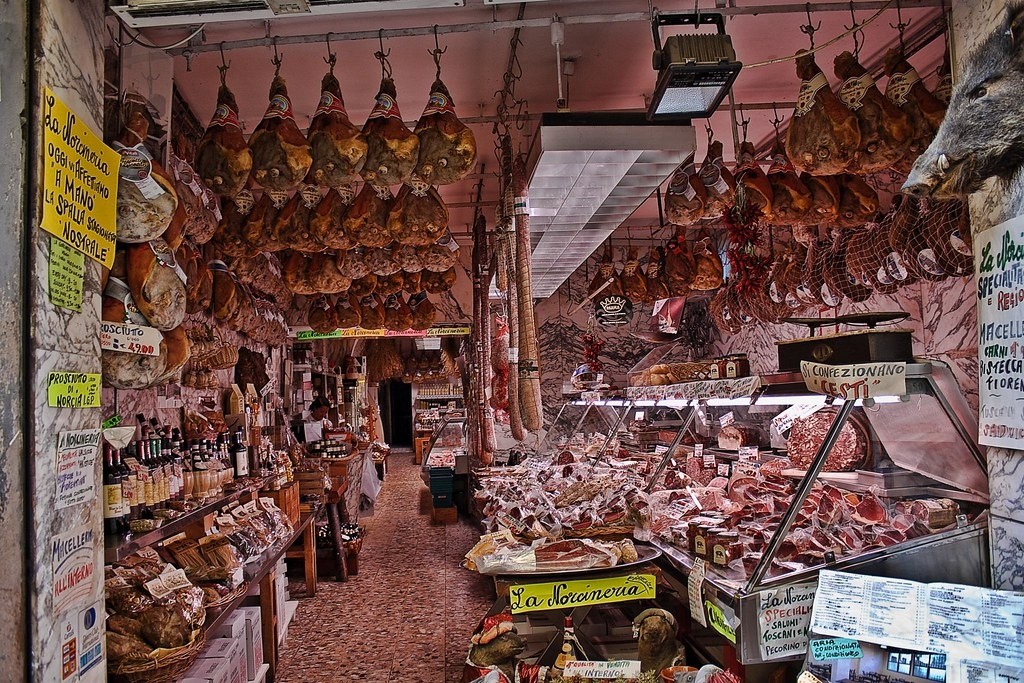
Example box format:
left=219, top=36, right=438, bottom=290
left=630, top=364, right=679, bottom=385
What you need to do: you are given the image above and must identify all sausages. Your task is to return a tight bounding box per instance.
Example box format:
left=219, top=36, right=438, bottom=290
left=471, top=148, right=544, bottom=464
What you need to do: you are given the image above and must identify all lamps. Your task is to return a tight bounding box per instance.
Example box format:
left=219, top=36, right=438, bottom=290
left=646, top=15, right=743, bottom=120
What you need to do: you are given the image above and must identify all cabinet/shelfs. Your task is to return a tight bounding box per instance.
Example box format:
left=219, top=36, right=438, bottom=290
left=104, top=471, right=317, bottom=683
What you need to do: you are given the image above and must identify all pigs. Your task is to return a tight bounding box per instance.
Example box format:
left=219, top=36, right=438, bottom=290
left=899, top=0, right=1024, bottom=200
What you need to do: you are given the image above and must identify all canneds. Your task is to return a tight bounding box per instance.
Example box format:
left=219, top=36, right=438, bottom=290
left=686, top=522, right=718, bottom=560
left=311, top=439, right=348, bottom=458
left=712, top=532, right=744, bottom=569
left=710, top=353, right=750, bottom=380
left=704, top=528, right=728, bottom=563
left=700, top=510, right=732, bottom=529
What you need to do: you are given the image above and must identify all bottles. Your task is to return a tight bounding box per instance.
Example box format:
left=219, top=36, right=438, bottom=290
left=318, top=523, right=359, bottom=541
left=419, top=384, right=448, bottom=395
left=550, top=616, right=578, bottom=673
left=257, top=445, right=293, bottom=491
left=103, top=412, right=250, bottom=548
left=244, top=402, right=250, bottom=413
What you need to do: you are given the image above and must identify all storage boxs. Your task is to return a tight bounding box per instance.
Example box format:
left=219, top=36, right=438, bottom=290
left=174, top=545, right=287, bottom=683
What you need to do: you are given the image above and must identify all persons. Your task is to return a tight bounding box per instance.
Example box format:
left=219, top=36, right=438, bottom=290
left=303, top=396, right=333, bottom=429
